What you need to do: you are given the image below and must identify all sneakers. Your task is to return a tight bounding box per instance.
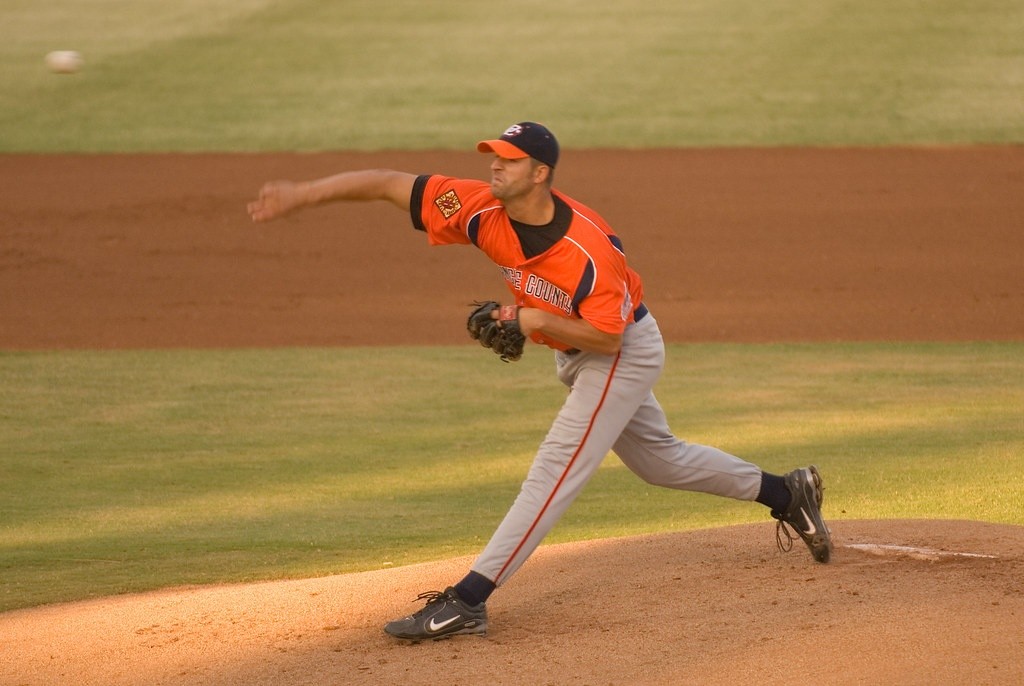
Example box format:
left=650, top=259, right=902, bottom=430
left=384, top=585, right=487, bottom=643
left=771, top=464, right=831, bottom=563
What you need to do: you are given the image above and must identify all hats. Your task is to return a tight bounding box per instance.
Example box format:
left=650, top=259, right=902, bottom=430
left=476, top=121, right=559, bottom=169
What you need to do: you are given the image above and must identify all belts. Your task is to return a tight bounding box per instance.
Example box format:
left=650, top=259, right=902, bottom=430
left=564, top=302, right=647, bottom=355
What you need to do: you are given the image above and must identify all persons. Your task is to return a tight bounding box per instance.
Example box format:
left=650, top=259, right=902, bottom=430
left=247, top=122, right=831, bottom=641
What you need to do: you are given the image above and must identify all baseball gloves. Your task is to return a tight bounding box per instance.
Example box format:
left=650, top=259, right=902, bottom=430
left=466, top=297, right=527, bottom=365
